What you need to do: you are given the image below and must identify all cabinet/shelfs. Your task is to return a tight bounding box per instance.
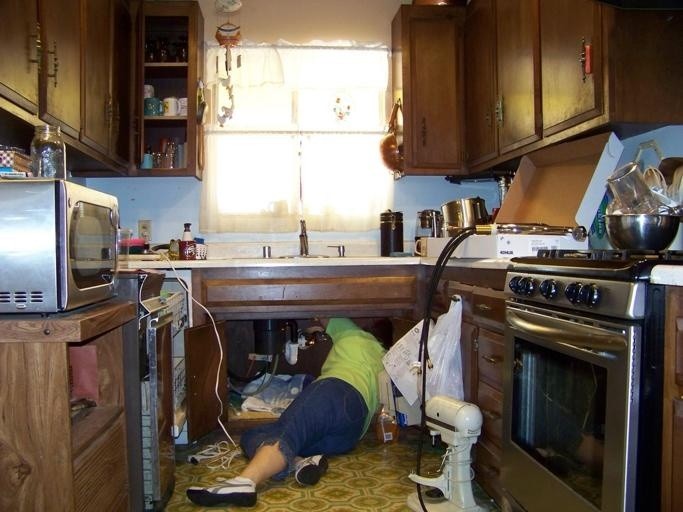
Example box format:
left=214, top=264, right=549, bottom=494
left=0, top=0, right=205, bottom=181
left=425, top=265, right=506, bottom=509
left=662, top=284, right=683, bottom=512
left=390, top=1, right=683, bottom=176
left=0, top=300, right=144, bottom=512
left=184, top=264, right=425, bottom=445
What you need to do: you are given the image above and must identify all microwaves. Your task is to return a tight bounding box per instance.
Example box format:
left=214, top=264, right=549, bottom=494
left=0, top=176, right=122, bottom=315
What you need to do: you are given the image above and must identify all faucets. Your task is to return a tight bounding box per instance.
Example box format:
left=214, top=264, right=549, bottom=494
left=299, top=220, right=309, bottom=256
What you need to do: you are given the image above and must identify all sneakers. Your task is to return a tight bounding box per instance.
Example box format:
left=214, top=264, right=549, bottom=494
left=185, top=478, right=257, bottom=506
left=294, top=454, right=327, bottom=487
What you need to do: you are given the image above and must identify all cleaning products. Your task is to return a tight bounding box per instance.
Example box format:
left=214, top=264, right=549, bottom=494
left=182, top=223, right=193, bottom=242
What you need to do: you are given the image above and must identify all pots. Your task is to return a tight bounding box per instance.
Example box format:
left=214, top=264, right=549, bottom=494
left=440, top=196, right=489, bottom=237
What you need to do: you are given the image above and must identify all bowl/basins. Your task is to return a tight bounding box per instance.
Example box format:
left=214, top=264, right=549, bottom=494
left=603, top=213, right=682, bottom=253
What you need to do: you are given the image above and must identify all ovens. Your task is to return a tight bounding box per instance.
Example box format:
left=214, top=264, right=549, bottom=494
left=497, top=296, right=663, bottom=511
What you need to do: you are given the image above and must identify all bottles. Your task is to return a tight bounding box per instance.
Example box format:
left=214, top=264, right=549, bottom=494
left=145, top=35, right=188, bottom=62
left=140, top=134, right=188, bottom=169
left=28, top=124, right=67, bottom=181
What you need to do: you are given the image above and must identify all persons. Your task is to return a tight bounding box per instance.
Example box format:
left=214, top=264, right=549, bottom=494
left=185, top=317, right=388, bottom=507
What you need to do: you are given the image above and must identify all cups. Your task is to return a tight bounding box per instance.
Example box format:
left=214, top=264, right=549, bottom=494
left=607, top=161, right=657, bottom=215
left=144, top=85, right=156, bottom=99
left=177, top=97, right=189, bottom=117
left=143, top=97, right=165, bottom=116
left=168, top=239, right=181, bottom=260
left=379, top=209, right=404, bottom=256
left=413, top=208, right=444, bottom=258
left=195, top=243, right=208, bottom=260
left=162, top=96, right=181, bottom=117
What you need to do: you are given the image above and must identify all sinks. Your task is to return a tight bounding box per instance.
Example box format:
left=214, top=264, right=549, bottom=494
left=272, top=253, right=330, bottom=258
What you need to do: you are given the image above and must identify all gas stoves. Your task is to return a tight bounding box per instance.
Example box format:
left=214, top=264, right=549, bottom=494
left=502, top=246, right=683, bottom=320
